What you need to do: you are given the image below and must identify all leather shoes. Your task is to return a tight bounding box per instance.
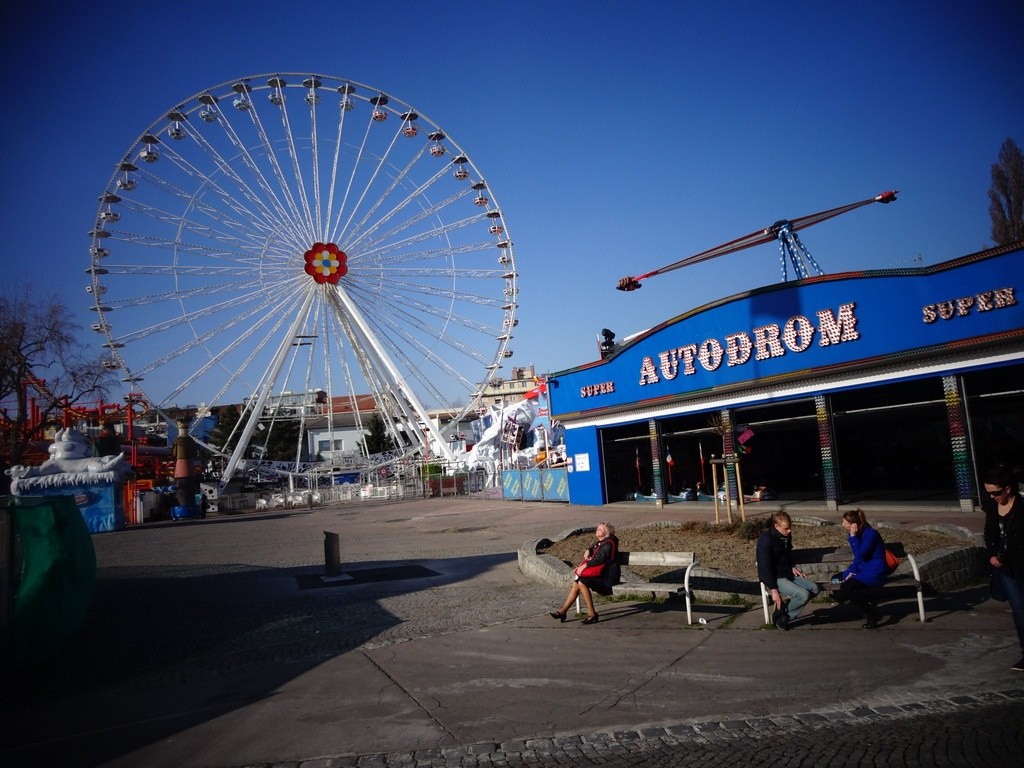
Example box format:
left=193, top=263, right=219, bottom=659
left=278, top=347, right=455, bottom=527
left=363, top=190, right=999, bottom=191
left=775, top=612, right=789, bottom=631
left=862, top=614, right=884, bottom=630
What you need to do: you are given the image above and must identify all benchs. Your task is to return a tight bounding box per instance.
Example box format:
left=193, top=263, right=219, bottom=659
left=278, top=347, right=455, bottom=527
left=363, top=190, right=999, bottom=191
left=753, top=554, right=927, bottom=625
left=575, top=551, right=697, bottom=624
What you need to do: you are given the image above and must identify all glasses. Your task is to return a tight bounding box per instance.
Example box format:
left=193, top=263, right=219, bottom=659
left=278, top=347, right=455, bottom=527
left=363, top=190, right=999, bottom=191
left=986, top=490, right=1005, bottom=495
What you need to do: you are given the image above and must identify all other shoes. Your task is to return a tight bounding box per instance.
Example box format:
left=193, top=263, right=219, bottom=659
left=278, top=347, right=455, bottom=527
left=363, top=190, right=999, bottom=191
left=1012, top=655, right=1024, bottom=671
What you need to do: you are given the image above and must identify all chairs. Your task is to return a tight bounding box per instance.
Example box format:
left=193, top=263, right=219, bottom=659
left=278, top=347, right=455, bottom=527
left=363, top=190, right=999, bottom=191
left=253, top=491, right=321, bottom=510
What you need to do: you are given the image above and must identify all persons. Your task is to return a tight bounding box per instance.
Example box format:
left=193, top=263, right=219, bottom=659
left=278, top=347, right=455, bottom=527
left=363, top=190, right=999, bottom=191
left=533, top=445, right=567, bottom=468
left=757, top=511, right=821, bottom=631
left=549, top=521, right=621, bottom=625
left=839, top=508, right=888, bottom=629
left=980, top=463, right=1024, bottom=672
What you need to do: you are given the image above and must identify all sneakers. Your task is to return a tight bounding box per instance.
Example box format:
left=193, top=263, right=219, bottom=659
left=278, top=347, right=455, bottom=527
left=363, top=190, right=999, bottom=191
left=770, top=601, right=785, bottom=624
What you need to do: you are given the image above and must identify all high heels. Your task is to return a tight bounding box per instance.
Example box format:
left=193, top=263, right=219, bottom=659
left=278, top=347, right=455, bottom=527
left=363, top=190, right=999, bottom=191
left=582, top=613, right=599, bottom=625
left=550, top=611, right=567, bottom=622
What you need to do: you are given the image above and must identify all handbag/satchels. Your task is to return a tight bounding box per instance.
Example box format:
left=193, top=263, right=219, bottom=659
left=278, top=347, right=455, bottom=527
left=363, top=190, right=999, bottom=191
left=885, top=550, right=899, bottom=568
left=989, top=567, right=1008, bottom=602
left=575, top=557, right=605, bottom=576
